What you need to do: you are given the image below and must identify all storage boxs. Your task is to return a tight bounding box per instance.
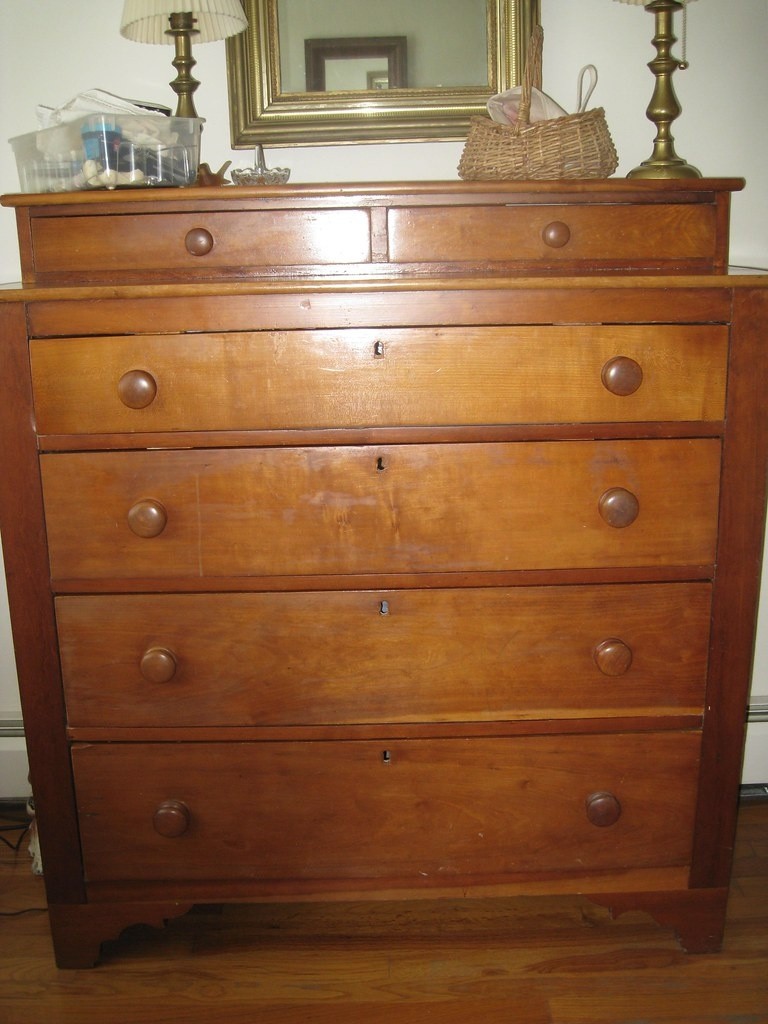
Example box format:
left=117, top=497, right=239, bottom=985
left=7, top=111, right=206, bottom=193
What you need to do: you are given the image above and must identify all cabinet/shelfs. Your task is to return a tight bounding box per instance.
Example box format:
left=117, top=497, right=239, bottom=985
left=0, top=175, right=768, bottom=967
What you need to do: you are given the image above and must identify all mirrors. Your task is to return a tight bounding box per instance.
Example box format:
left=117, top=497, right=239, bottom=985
left=225, top=0, right=542, bottom=151
left=304, top=35, right=408, bottom=93
left=367, top=71, right=388, bottom=89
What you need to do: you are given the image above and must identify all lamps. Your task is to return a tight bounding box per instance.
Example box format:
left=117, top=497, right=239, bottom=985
left=121, top=0, right=249, bottom=185
left=614, top=0, right=704, bottom=178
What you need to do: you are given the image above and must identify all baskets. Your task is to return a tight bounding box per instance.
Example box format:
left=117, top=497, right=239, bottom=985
left=458, top=24, right=618, bottom=181
